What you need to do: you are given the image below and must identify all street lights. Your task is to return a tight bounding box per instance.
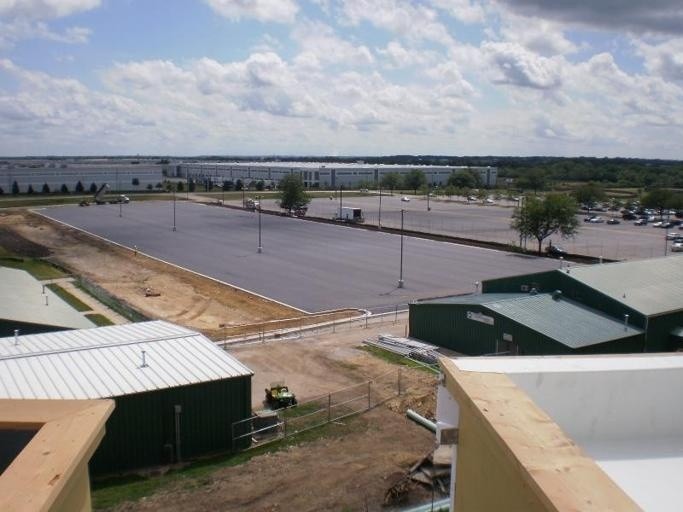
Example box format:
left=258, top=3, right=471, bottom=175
left=240, top=185, right=248, bottom=209
left=220, top=181, right=224, bottom=206
left=339, top=184, right=344, bottom=225
left=255, top=195, right=264, bottom=253
left=172, top=185, right=177, bottom=231
left=375, top=183, right=385, bottom=230
left=398, top=208, right=409, bottom=288
left=118, top=176, right=122, bottom=217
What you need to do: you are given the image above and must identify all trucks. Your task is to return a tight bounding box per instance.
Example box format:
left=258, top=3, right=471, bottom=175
left=331, top=206, right=362, bottom=224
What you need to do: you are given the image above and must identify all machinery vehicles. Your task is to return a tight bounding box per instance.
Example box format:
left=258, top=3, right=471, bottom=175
left=243, top=197, right=259, bottom=210
left=93, top=183, right=129, bottom=205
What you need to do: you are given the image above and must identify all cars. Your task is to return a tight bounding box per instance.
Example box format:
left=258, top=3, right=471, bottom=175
left=400, top=196, right=409, bottom=201
left=427, top=185, right=542, bottom=204
left=580, top=197, right=682, bottom=252
left=79, top=199, right=88, bottom=206
left=543, top=245, right=568, bottom=255
left=359, top=188, right=368, bottom=192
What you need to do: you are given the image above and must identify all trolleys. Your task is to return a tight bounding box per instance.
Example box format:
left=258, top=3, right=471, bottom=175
left=381, top=469, right=421, bottom=506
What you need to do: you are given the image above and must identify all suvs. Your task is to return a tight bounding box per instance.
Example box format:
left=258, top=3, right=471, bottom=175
left=264, top=380, right=296, bottom=410
